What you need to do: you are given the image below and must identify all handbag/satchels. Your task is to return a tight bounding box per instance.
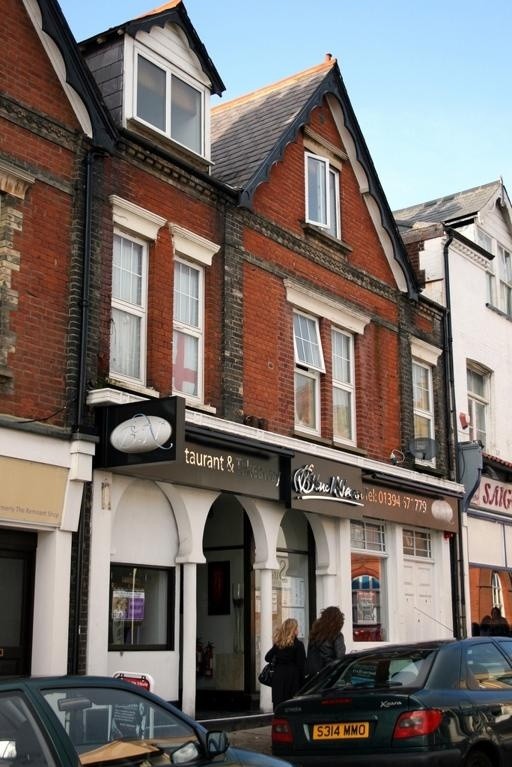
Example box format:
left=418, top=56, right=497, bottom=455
left=258, top=660, right=274, bottom=688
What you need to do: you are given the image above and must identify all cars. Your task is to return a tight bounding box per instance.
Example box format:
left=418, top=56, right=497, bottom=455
left=0, top=675, right=295, bottom=766
left=271, top=635, right=511, bottom=766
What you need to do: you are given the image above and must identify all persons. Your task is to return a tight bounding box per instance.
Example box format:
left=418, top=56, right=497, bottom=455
left=265, top=619, right=305, bottom=707
left=477, top=615, right=488, bottom=635
left=305, top=605, right=346, bottom=673
left=483, top=607, right=512, bottom=638
left=470, top=623, right=480, bottom=635
